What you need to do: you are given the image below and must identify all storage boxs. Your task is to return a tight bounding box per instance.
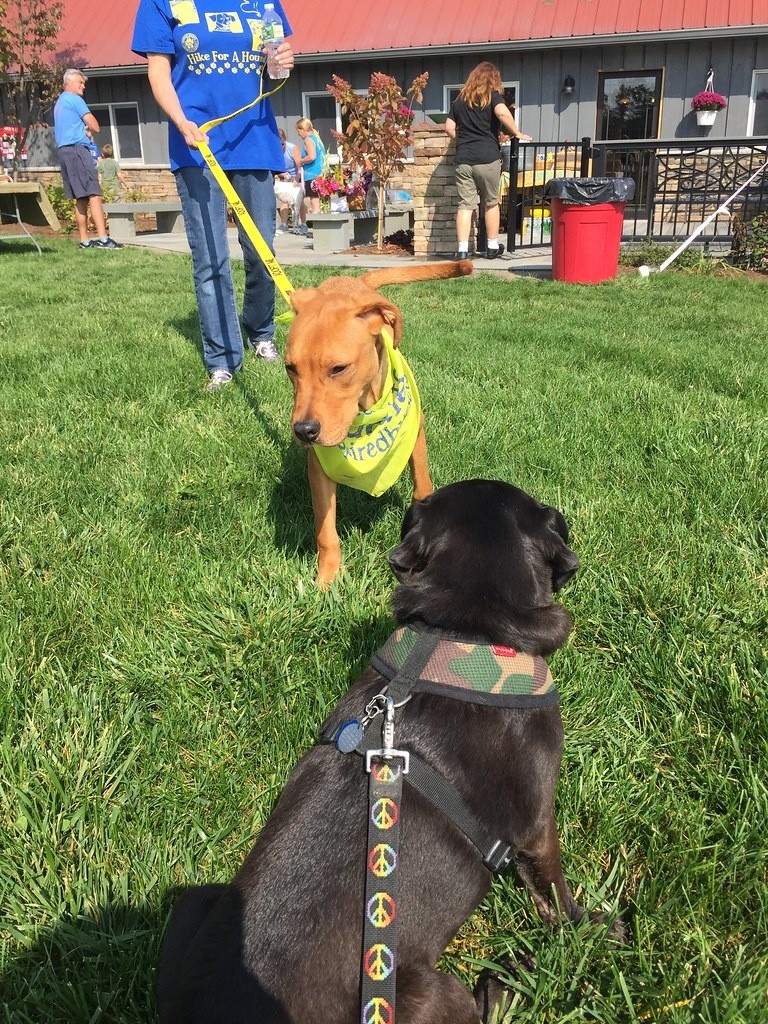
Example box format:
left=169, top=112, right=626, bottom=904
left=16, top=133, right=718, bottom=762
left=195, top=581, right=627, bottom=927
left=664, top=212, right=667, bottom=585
left=524, top=218, right=552, bottom=235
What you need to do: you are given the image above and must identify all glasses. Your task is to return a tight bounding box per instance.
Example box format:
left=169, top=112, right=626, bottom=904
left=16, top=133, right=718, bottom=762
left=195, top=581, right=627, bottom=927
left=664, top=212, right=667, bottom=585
left=282, top=139, right=285, bottom=146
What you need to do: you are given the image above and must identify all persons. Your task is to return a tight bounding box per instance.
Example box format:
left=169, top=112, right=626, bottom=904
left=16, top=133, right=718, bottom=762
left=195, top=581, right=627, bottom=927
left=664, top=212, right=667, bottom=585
left=96, top=144, right=130, bottom=189
left=296, top=118, right=326, bottom=212
left=54, top=69, right=125, bottom=250
left=132, top=0, right=294, bottom=392
left=445, top=61, right=532, bottom=261
left=275, top=128, right=308, bottom=235
left=85, top=126, right=102, bottom=182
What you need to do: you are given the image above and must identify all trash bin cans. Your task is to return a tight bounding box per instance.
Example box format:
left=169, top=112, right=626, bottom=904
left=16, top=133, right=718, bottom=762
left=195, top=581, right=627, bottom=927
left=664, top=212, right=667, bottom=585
left=549, top=177, right=633, bottom=284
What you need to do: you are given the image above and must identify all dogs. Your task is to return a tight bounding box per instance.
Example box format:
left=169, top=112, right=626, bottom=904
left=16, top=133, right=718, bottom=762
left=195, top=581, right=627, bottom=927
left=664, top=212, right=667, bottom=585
left=284, top=260, right=475, bottom=587
left=153, top=477, right=612, bottom=1024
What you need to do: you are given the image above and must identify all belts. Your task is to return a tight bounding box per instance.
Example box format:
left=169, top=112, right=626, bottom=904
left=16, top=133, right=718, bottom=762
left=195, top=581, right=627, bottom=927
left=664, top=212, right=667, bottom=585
left=59, top=144, right=90, bottom=152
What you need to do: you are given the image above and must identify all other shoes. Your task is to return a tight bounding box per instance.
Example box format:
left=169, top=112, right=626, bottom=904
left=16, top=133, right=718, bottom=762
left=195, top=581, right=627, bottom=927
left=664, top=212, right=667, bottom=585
left=454, top=251, right=468, bottom=260
left=487, top=243, right=505, bottom=259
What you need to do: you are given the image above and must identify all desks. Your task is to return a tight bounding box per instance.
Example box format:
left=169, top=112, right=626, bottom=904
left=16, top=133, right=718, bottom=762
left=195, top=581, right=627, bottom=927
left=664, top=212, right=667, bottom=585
left=498, top=170, right=581, bottom=234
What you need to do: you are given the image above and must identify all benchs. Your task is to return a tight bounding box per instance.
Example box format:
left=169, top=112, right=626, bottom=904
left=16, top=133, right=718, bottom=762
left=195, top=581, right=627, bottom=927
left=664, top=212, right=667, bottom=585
left=101, top=202, right=186, bottom=237
left=384, top=200, right=414, bottom=241
left=305, top=210, right=389, bottom=252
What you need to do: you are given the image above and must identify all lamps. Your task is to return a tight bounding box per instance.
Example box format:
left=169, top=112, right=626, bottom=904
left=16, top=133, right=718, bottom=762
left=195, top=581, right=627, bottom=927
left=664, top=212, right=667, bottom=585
left=561, top=75, right=576, bottom=95
left=619, top=98, right=630, bottom=104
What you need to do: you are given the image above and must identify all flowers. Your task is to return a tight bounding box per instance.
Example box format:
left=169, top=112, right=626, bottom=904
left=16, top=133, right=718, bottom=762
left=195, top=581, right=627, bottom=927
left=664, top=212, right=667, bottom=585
left=308, top=143, right=373, bottom=197
left=691, top=91, right=727, bottom=112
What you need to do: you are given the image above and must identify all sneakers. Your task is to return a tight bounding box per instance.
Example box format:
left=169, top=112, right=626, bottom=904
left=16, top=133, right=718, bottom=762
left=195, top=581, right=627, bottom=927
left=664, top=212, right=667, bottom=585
left=276, top=225, right=288, bottom=234
left=94, top=236, right=125, bottom=249
left=247, top=335, right=279, bottom=360
left=299, top=224, right=308, bottom=236
left=79, top=239, right=95, bottom=249
left=207, top=370, right=234, bottom=390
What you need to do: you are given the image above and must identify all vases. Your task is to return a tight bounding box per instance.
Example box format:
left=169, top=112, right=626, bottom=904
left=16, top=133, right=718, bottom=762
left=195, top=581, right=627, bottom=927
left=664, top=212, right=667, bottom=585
left=318, top=191, right=366, bottom=213
left=695, top=111, right=718, bottom=126
